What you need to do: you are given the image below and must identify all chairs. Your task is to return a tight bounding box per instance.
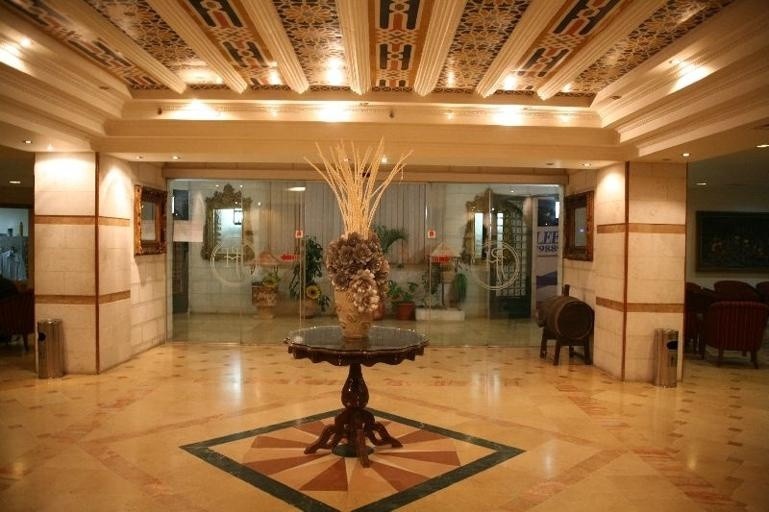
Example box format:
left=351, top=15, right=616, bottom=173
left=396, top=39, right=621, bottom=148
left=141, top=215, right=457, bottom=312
left=684, top=280, right=769, bottom=369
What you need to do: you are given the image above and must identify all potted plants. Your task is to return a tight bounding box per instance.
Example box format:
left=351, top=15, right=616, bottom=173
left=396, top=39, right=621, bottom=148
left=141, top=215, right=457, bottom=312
left=386, top=279, right=420, bottom=320
left=250, top=271, right=282, bottom=320
left=287, top=235, right=324, bottom=318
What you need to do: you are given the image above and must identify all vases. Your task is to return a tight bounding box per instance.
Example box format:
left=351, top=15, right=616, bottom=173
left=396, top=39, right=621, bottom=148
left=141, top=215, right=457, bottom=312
left=326, top=290, right=390, bottom=341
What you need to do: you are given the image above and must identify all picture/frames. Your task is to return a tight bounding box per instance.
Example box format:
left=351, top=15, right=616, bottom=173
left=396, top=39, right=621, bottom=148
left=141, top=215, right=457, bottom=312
left=695, top=210, right=769, bottom=273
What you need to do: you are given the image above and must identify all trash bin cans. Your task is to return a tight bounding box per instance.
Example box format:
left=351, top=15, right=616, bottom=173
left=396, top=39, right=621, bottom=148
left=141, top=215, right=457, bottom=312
left=653, top=328, right=679, bottom=388
left=37, top=318, right=64, bottom=378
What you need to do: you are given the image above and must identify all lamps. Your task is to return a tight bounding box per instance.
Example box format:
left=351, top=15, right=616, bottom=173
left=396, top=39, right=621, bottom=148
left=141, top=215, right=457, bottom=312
left=250, top=180, right=282, bottom=267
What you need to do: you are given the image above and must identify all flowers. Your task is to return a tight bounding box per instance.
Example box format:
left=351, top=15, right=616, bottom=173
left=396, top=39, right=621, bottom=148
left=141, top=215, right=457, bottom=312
left=302, top=135, right=415, bottom=315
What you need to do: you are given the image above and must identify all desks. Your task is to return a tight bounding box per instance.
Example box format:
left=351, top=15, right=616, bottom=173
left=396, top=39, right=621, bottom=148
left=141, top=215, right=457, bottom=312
left=282, top=323, right=431, bottom=468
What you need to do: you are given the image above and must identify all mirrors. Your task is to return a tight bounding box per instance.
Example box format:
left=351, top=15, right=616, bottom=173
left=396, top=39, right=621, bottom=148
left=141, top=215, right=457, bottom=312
left=200, top=181, right=254, bottom=263
left=461, top=190, right=514, bottom=266
left=562, top=190, right=595, bottom=263
left=134, top=183, right=170, bottom=257
